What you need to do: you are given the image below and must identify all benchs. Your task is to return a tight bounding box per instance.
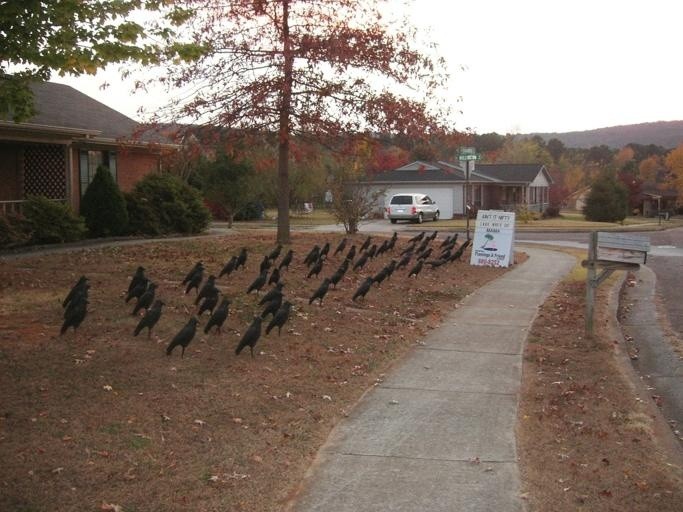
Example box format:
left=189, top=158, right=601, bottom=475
left=597, top=232, right=650, bottom=265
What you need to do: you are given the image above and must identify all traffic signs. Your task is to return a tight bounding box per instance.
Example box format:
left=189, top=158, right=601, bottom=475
left=456, top=147, right=477, bottom=155
left=458, top=153, right=481, bottom=161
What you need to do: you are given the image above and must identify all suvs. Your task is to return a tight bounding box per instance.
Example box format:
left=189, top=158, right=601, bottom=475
left=385, top=194, right=440, bottom=224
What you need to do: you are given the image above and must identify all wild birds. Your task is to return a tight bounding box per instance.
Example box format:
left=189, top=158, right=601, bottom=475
left=236, top=317, right=265, bottom=357
left=166, top=317, right=201, bottom=360
left=60, top=231, right=472, bottom=338
